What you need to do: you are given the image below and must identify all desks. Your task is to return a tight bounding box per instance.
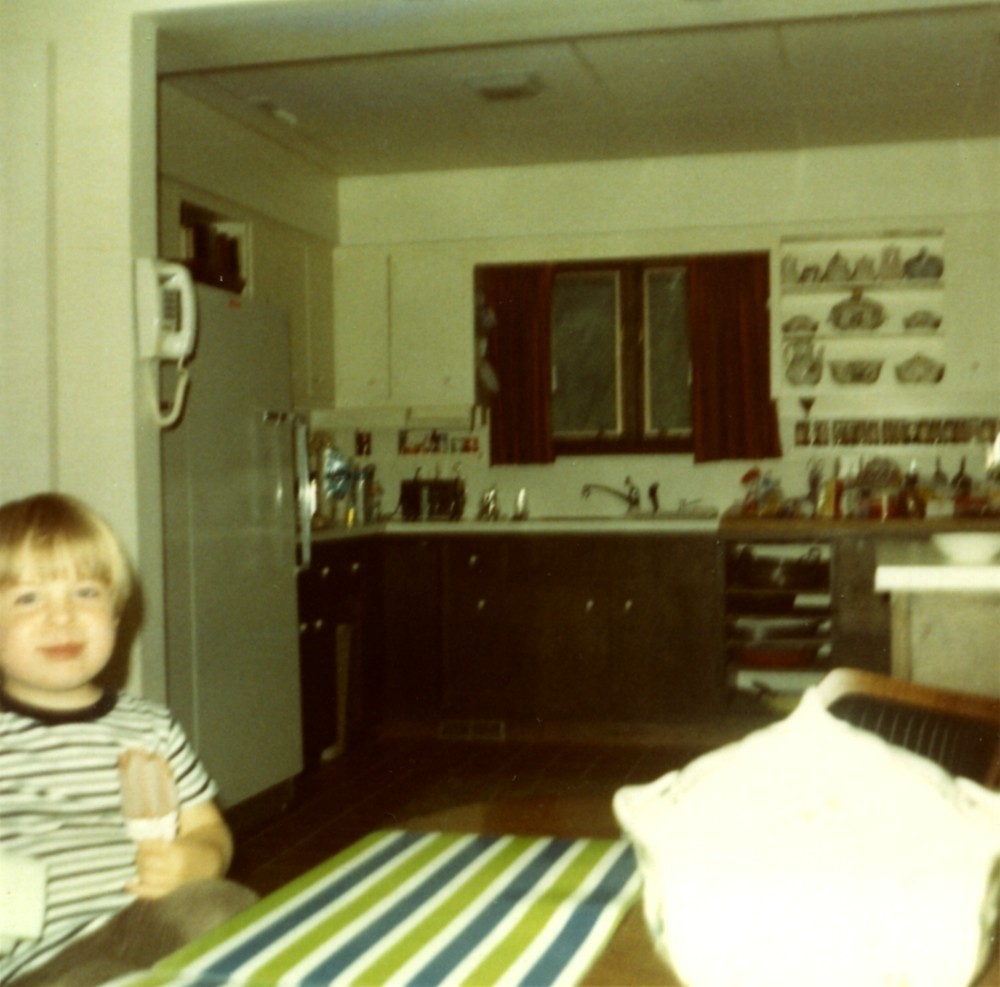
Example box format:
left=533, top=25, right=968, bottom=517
left=100, top=829, right=678, bottom=987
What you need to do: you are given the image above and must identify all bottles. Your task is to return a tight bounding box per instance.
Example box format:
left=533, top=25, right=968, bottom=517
left=308, top=429, right=376, bottom=530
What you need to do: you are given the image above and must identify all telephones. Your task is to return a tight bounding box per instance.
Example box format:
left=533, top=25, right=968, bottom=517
left=135, top=257, right=197, bottom=360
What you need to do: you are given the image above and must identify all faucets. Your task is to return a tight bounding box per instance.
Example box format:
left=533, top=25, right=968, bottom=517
left=581, top=475, right=642, bottom=515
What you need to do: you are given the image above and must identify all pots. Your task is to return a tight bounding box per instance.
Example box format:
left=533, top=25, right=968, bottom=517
left=750, top=548, right=829, bottom=590
left=829, top=290, right=885, bottom=330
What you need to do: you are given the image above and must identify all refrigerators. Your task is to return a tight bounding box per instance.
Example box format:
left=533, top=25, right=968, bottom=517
left=163, top=282, right=303, bottom=811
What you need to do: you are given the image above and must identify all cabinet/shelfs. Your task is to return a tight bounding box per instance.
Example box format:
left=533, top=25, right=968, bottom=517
left=295, top=540, right=724, bottom=768
left=777, top=229, right=950, bottom=398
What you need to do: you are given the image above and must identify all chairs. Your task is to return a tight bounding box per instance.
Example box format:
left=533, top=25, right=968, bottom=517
left=794, top=666, right=1000, bottom=788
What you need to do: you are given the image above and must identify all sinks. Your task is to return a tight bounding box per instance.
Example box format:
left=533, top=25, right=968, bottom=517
left=543, top=515, right=654, bottom=521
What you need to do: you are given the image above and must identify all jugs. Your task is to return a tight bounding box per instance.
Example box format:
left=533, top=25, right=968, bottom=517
left=783, top=344, right=826, bottom=386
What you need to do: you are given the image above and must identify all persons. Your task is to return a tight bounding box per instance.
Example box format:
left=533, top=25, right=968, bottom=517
left=0, top=491, right=255, bottom=987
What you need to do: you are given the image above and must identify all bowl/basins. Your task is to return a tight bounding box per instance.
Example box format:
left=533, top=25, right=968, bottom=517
left=831, top=360, right=882, bottom=384
left=897, top=351, right=944, bottom=381
